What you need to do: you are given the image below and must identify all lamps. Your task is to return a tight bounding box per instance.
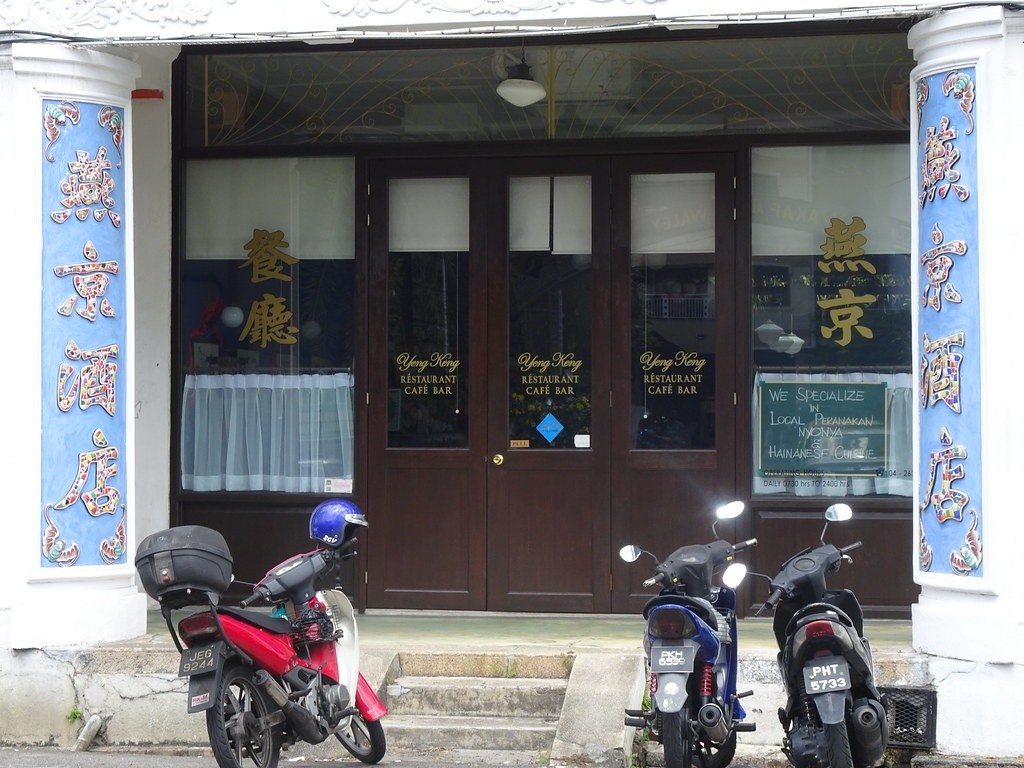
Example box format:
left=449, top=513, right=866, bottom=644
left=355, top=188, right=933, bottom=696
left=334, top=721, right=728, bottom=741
left=753, top=297, right=806, bottom=359
left=496, top=37, right=546, bottom=111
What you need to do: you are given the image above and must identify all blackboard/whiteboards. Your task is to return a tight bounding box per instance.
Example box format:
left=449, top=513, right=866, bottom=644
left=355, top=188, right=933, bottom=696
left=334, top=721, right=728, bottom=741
left=757, top=380, right=888, bottom=478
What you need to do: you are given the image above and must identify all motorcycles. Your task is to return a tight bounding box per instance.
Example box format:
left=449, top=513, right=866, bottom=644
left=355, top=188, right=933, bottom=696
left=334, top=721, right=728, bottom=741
left=588, top=500, right=762, bottom=768
left=137, top=526, right=391, bottom=768
left=721, top=505, right=896, bottom=768
